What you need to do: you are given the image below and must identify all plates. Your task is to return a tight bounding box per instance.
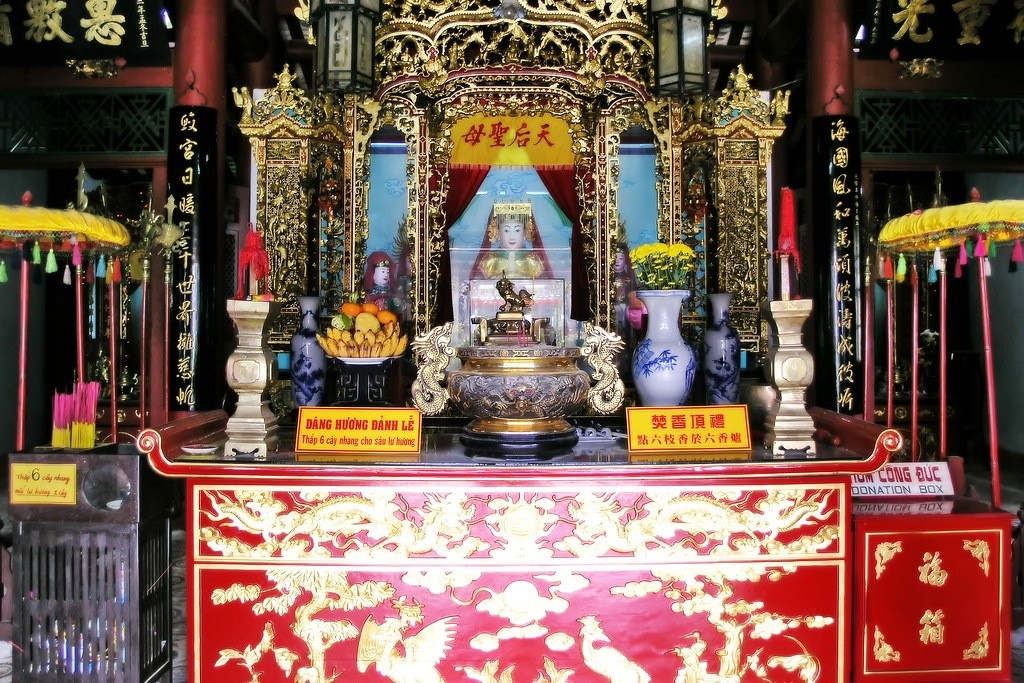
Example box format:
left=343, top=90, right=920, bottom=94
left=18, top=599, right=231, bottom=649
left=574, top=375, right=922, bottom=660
left=182, top=445, right=219, bottom=455
left=327, top=356, right=403, bottom=365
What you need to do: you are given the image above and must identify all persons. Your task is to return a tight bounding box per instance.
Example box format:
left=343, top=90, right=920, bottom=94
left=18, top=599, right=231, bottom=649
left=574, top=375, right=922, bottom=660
left=474, top=202, right=550, bottom=279
left=613, top=242, right=638, bottom=330
left=364, top=252, right=394, bottom=310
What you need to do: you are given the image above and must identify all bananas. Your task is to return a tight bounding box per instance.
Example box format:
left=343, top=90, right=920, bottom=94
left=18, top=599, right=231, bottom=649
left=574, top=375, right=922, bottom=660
left=315, top=320, right=408, bottom=357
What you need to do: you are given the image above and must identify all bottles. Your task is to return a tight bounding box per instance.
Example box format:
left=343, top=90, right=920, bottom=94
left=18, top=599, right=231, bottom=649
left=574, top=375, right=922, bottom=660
left=702, top=293, right=740, bottom=405
left=814, top=426, right=841, bottom=446
left=291, top=295, right=324, bottom=412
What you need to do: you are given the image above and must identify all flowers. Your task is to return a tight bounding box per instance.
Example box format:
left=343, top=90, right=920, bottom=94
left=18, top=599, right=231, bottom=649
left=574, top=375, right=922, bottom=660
left=629, top=242, right=698, bottom=291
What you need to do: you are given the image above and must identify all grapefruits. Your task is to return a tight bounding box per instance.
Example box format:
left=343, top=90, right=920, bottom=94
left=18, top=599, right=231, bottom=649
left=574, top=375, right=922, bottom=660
left=355, top=312, right=381, bottom=334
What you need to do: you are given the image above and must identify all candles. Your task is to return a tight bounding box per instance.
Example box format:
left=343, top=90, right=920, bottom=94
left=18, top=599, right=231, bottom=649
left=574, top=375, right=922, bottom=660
left=237, top=221, right=269, bottom=301
left=779, top=186, right=800, bottom=273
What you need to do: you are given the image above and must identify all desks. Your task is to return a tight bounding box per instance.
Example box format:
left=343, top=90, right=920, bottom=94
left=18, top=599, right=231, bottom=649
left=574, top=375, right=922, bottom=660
left=136, top=406, right=906, bottom=683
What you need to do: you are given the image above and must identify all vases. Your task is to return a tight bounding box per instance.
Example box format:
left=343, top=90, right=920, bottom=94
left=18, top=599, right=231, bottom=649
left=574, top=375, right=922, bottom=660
left=702, top=292, right=741, bottom=406
left=631, top=290, right=695, bottom=408
left=291, top=296, right=326, bottom=411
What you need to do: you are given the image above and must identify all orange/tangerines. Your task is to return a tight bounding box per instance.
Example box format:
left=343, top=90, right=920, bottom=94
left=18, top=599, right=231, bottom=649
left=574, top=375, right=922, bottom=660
left=361, top=303, right=378, bottom=315
left=342, top=302, right=362, bottom=317
left=377, top=310, right=398, bottom=324
